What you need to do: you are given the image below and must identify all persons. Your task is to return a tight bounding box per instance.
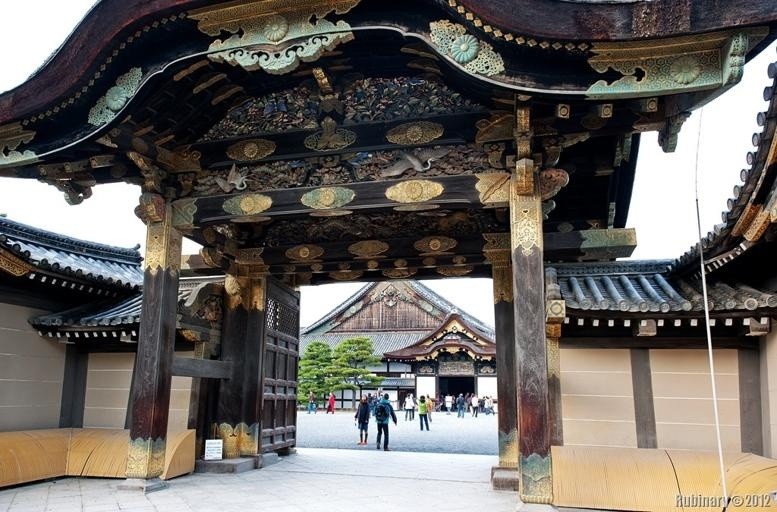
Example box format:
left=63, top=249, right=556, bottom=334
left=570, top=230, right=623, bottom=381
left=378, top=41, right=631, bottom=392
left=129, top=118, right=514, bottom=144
left=324, top=391, right=336, bottom=414
left=371, top=393, right=397, bottom=450
left=415, top=394, right=430, bottom=431
left=402, top=392, right=495, bottom=422
left=305, top=389, right=317, bottom=414
left=365, top=391, right=384, bottom=416
left=353, top=394, right=371, bottom=445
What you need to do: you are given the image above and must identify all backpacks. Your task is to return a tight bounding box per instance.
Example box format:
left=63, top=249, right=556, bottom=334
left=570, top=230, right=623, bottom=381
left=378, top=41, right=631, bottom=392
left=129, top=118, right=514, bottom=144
left=375, top=404, right=390, bottom=422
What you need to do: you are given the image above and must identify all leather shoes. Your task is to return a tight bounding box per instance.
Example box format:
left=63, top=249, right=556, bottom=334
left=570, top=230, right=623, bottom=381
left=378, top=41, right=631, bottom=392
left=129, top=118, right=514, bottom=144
left=358, top=440, right=392, bottom=451
